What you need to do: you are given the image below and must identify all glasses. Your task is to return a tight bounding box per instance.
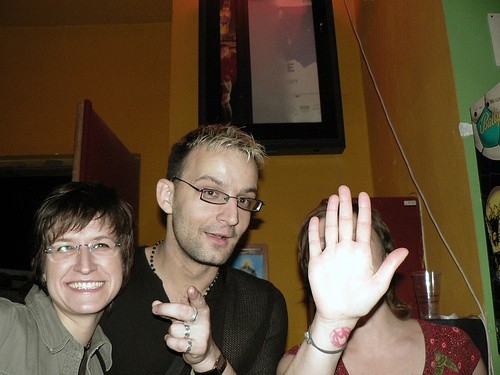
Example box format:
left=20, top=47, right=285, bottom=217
left=42, top=238, right=126, bottom=258
left=171, top=176, right=264, bottom=212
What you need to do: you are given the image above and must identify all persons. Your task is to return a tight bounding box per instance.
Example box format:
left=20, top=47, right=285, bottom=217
left=98, top=125, right=289, bottom=375
left=0, top=182, right=135, bottom=375
left=276, top=186, right=487, bottom=375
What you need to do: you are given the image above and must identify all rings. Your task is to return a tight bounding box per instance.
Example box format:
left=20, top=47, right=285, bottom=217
left=188, top=305, right=198, bottom=322
left=182, top=340, right=192, bottom=353
left=182, top=323, right=190, bottom=339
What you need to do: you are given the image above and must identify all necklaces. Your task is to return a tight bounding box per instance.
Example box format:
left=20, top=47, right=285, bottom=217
left=151, top=240, right=220, bottom=298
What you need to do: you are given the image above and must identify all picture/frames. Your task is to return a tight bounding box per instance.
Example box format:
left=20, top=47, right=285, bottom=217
left=198, top=0, right=346, bottom=156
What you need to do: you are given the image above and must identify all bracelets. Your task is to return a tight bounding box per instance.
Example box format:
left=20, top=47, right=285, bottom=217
left=304, top=327, right=348, bottom=354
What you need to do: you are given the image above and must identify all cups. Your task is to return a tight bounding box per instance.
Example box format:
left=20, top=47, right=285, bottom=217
left=412, top=270, right=441, bottom=320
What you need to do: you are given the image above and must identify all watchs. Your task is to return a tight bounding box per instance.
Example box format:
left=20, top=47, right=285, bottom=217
left=193, top=351, right=227, bottom=375
